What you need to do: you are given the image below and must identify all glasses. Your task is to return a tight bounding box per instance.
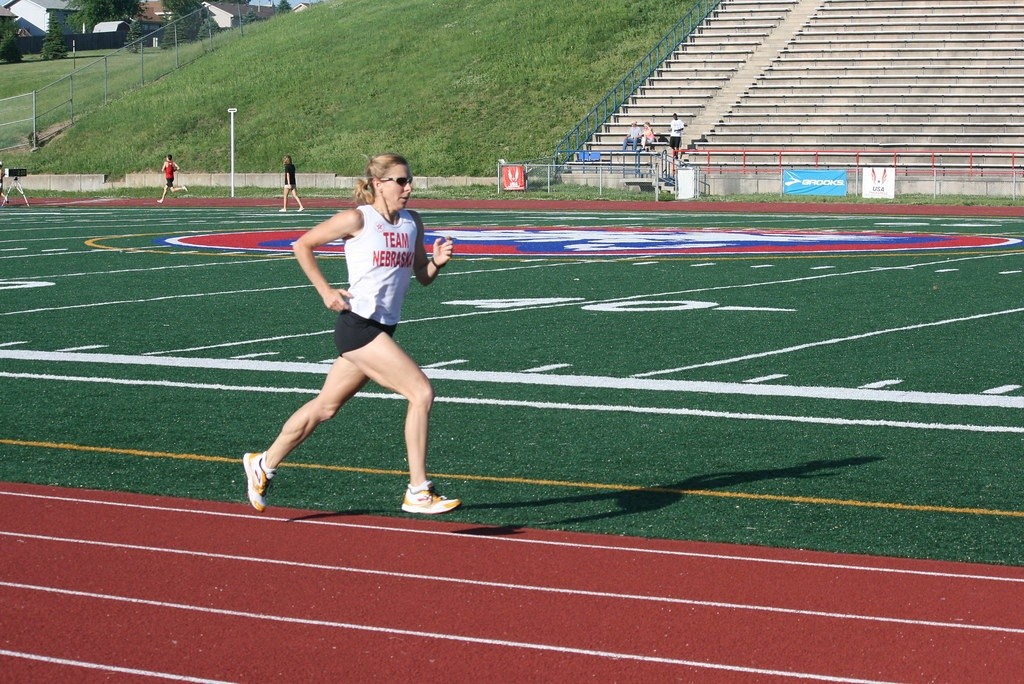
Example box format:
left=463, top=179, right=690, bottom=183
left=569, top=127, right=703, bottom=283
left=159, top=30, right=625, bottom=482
left=381, top=177, right=413, bottom=187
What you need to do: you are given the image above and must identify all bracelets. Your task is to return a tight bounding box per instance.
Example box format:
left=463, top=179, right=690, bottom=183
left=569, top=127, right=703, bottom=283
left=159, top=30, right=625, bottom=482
left=431, top=257, right=441, bottom=270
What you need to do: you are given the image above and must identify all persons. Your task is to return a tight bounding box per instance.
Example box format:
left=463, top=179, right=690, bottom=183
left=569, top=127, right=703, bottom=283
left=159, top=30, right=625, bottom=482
left=623, top=120, right=655, bottom=150
left=279, top=155, right=304, bottom=212
left=157, top=154, right=188, bottom=203
left=243, top=152, right=461, bottom=515
left=668, top=113, right=684, bottom=157
left=0, top=161, right=9, bottom=204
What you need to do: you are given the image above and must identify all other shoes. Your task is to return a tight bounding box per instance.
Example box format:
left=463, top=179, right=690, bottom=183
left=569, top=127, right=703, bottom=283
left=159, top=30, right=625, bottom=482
left=157, top=199, right=163, bottom=203
left=183, top=186, right=189, bottom=193
left=297, top=208, right=304, bottom=212
left=279, top=209, right=287, bottom=213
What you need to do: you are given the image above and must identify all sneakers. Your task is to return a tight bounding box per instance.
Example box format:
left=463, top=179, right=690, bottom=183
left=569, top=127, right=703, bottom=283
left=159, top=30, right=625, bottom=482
left=243, top=452, right=277, bottom=513
left=401, top=481, right=462, bottom=513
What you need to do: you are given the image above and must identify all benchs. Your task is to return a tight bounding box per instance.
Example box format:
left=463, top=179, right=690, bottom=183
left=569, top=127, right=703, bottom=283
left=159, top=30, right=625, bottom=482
left=565, top=0, right=1024, bottom=177
